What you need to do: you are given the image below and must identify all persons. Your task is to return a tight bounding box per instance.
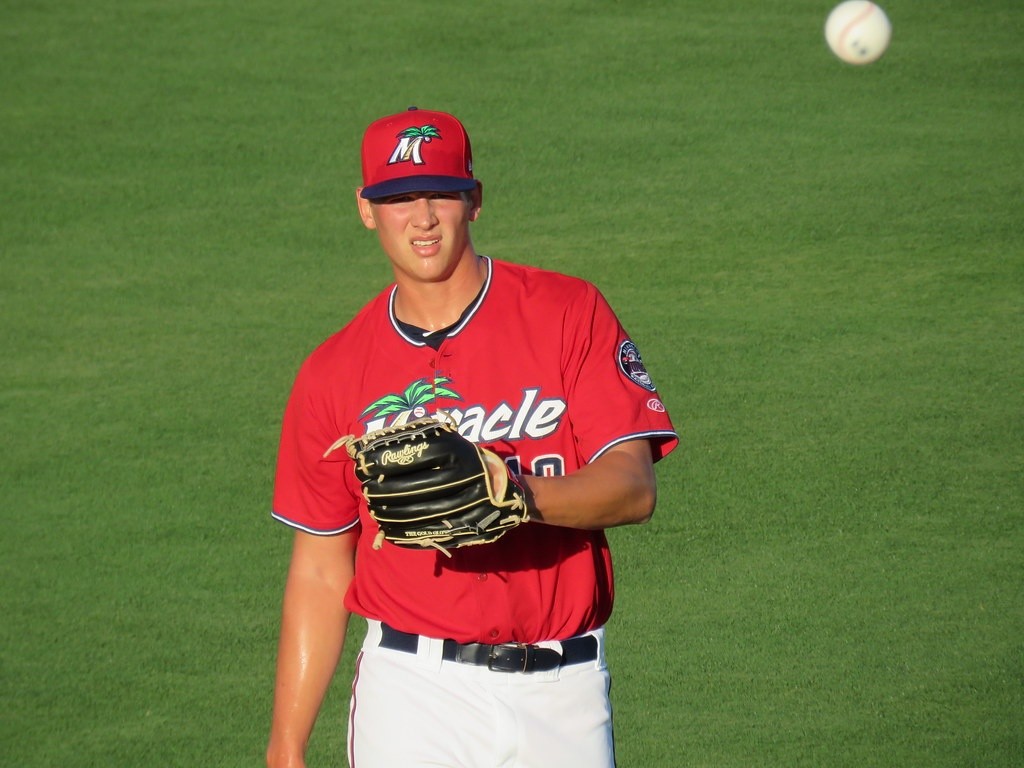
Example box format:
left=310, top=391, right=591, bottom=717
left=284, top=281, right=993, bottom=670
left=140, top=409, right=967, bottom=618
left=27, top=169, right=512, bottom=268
left=266, top=106, right=679, bottom=768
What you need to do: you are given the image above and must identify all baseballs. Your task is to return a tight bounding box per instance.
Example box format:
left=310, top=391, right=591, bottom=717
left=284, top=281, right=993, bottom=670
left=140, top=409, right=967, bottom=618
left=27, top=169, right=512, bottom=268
left=823, top=0, right=894, bottom=65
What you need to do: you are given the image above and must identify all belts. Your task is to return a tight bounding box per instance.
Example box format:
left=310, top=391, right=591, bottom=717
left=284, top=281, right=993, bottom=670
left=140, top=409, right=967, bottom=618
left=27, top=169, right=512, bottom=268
left=379, top=623, right=598, bottom=672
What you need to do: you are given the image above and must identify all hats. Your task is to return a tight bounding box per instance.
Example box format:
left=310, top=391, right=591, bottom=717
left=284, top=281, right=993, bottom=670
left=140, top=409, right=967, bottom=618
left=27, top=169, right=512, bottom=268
left=359, top=106, right=477, bottom=198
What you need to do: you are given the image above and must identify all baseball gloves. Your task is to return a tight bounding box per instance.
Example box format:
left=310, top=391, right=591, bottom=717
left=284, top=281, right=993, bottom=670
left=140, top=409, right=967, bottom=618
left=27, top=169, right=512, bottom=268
left=347, top=417, right=530, bottom=552
